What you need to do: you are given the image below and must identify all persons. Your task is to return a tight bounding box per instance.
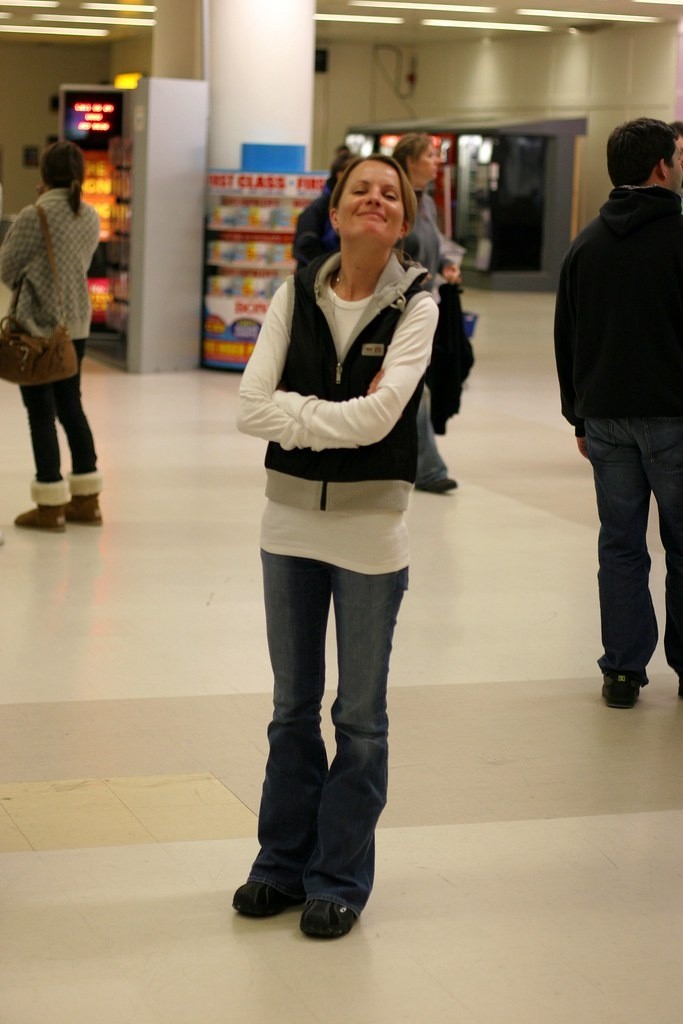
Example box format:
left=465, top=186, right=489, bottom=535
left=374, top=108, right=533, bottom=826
left=233, top=156, right=438, bottom=940
left=3, top=140, right=104, bottom=535
left=553, top=117, right=683, bottom=711
left=293, top=132, right=475, bottom=494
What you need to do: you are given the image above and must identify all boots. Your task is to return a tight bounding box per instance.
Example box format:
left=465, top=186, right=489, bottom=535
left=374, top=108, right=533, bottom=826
left=14, top=479, right=73, bottom=534
left=65, top=471, right=103, bottom=526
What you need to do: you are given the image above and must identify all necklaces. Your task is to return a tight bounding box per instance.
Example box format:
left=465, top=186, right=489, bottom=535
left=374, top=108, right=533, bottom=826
left=332, top=272, right=337, bottom=342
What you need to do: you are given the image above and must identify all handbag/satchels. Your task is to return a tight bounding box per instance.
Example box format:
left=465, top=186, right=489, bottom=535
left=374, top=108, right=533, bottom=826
left=0, top=317, right=78, bottom=385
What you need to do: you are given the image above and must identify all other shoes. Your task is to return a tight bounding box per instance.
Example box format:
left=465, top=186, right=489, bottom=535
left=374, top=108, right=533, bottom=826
left=232, top=881, right=306, bottom=918
left=602, top=672, right=640, bottom=708
left=416, top=477, right=456, bottom=494
left=300, top=898, right=356, bottom=940
